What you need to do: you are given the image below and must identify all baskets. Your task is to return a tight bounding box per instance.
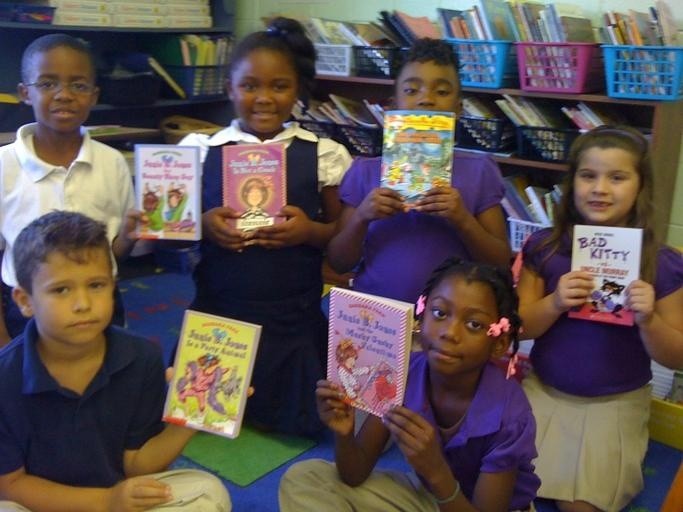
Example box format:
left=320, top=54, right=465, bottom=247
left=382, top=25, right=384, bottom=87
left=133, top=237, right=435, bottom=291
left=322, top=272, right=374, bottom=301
left=444, top=37, right=683, bottom=102
left=506, top=204, right=554, bottom=252
left=293, top=119, right=383, bottom=159
left=457, top=114, right=579, bottom=164
left=312, top=42, right=411, bottom=81
left=159, top=114, right=225, bottom=144
left=161, top=63, right=229, bottom=99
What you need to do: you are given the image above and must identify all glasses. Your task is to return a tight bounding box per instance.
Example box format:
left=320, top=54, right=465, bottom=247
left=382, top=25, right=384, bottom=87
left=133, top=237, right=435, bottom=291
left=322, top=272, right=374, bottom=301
left=24, top=78, right=96, bottom=97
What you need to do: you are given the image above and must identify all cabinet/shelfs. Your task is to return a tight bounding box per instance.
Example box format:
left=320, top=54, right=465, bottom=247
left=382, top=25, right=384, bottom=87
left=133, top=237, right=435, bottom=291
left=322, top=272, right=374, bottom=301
left=0, top=20, right=236, bottom=154
left=285, top=73, right=683, bottom=281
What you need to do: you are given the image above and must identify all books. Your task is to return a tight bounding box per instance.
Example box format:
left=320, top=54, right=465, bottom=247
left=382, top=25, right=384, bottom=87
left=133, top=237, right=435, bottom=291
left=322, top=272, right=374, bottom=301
left=498, top=164, right=683, bottom=411
left=323, top=286, right=417, bottom=421
left=155, top=306, right=266, bottom=440
left=377, top=104, right=458, bottom=214
left=130, top=138, right=206, bottom=246
left=87, top=1, right=682, bottom=161
left=216, top=141, right=290, bottom=251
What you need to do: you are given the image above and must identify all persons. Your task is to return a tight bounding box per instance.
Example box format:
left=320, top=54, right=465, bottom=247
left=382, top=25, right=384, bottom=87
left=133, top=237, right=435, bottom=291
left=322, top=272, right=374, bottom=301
left=0, top=213, right=229, bottom=511
left=0, top=34, right=150, bottom=340
left=516, top=124, right=681, bottom=512
left=279, top=258, right=540, bottom=512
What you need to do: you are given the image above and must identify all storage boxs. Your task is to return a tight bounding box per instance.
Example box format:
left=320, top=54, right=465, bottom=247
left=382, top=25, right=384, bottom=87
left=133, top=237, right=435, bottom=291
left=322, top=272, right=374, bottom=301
left=646, top=372, right=683, bottom=451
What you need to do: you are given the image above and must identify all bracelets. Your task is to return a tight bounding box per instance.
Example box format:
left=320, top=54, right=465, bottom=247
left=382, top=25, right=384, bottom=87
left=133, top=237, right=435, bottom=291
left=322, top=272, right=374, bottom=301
left=434, top=478, right=460, bottom=506
